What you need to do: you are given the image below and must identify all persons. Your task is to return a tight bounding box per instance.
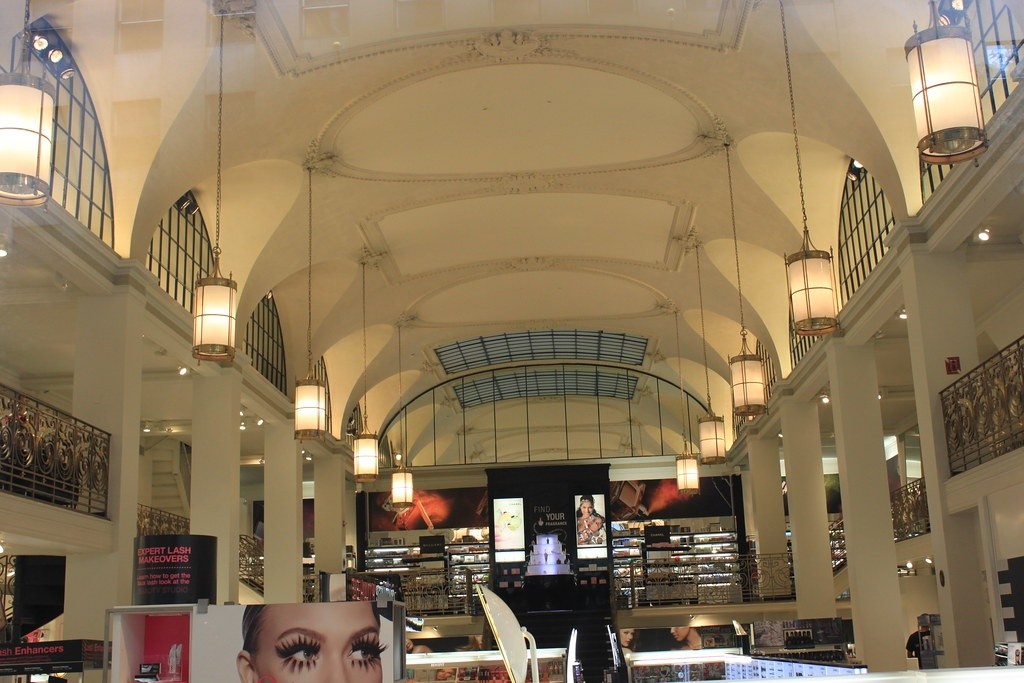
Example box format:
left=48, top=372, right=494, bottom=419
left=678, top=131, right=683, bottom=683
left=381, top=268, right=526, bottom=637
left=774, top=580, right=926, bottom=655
left=406, top=638, right=433, bottom=653
left=618, top=628, right=634, bottom=655
left=576, top=494, right=606, bottom=546
left=905, top=612, right=938, bottom=670
left=236, top=600, right=389, bottom=683
left=434, top=668, right=455, bottom=680
left=669, top=627, right=702, bottom=650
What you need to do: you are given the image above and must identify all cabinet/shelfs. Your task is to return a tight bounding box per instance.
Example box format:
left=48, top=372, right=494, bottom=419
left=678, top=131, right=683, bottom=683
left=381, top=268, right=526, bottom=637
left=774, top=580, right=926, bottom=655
left=610, top=535, right=742, bottom=603
left=364, top=543, right=488, bottom=610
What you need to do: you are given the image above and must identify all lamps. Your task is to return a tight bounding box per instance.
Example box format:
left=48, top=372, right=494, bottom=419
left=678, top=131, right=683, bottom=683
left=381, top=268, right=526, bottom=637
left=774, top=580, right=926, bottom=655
left=142, top=422, right=150, bottom=432
left=846, top=159, right=863, bottom=180
left=779, top=0, right=839, bottom=335
left=391, top=323, right=414, bottom=508
left=979, top=227, right=991, bottom=240
left=699, top=246, right=726, bottom=465
left=185, top=203, right=199, bottom=214
left=353, top=262, right=378, bottom=484
left=192, top=0, right=239, bottom=361
left=175, top=197, right=191, bottom=210
left=176, top=365, right=187, bottom=376
left=728, top=143, right=766, bottom=416
left=675, top=311, right=699, bottom=495
left=904, top=0, right=991, bottom=163
left=295, top=156, right=326, bottom=441
left=0, top=0, right=74, bottom=205
